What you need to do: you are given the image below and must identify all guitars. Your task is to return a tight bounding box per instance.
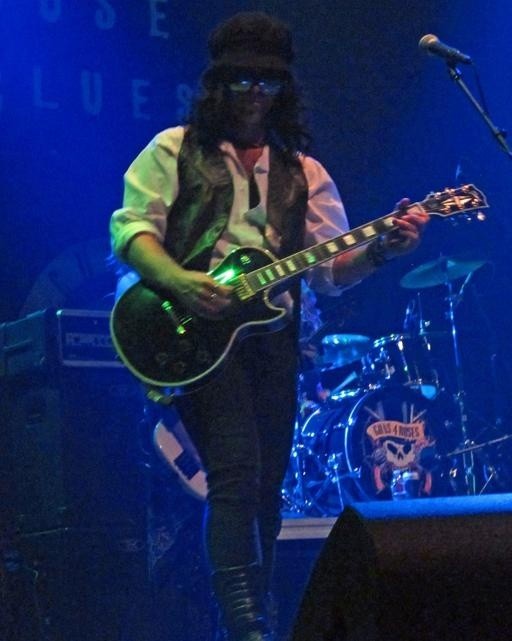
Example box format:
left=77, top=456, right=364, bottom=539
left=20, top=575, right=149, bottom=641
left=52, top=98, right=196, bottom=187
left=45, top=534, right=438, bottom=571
left=108, top=184, right=491, bottom=398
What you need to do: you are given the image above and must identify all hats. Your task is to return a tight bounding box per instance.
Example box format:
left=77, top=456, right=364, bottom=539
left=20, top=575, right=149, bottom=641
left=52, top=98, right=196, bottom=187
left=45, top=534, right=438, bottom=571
left=210, top=10, right=295, bottom=71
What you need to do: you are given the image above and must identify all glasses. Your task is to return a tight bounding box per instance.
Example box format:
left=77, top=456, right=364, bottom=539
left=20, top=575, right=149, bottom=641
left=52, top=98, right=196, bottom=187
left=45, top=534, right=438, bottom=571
left=222, top=76, right=283, bottom=96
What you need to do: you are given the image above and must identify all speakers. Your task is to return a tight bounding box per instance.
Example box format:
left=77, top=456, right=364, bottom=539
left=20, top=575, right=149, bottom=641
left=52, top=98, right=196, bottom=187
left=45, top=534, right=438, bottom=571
left=288, top=492, right=512, bottom=641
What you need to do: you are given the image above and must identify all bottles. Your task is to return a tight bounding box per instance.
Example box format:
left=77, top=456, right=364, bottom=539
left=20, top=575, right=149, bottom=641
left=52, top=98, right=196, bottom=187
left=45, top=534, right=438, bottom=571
left=390, top=469, right=410, bottom=501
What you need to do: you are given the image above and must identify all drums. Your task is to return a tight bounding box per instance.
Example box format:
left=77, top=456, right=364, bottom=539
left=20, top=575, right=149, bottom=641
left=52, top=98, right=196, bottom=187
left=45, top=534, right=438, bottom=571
left=365, top=332, right=438, bottom=400
left=299, top=385, right=448, bottom=500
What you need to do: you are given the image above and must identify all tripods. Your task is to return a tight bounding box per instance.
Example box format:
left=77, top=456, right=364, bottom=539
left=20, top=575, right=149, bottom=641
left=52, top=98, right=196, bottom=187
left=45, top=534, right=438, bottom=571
left=283, top=380, right=332, bottom=522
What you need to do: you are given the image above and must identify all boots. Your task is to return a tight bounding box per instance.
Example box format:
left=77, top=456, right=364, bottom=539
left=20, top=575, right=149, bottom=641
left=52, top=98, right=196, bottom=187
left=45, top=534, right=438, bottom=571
left=212, top=561, right=278, bottom=641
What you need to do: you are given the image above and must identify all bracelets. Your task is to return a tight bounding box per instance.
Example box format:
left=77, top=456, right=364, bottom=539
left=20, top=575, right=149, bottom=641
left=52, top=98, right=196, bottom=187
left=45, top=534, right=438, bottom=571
left=366, top=236, right=393, bottom=267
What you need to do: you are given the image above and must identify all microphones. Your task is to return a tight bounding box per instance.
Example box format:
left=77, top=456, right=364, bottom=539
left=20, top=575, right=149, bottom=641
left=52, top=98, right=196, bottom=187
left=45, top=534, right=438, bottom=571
left=419, top=31, right=472, bottom=73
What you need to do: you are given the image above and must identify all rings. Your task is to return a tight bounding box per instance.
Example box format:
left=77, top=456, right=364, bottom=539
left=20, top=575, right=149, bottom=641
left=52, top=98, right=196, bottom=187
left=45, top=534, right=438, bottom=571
left=208, top=291, right=218, bottom=305
left=210, top=282, right=220, bottom=291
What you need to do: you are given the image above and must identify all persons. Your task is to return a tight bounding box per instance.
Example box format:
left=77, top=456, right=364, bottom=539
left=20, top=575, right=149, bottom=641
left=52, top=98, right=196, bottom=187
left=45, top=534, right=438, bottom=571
left=107, top=12, right=429, bottom=641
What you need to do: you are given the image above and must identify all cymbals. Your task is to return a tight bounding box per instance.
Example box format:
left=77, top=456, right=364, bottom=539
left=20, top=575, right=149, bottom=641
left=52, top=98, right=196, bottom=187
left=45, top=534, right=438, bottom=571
left=400, top=252, right=489, bottom=290
left=298, top=333, right=371, bottom=372
